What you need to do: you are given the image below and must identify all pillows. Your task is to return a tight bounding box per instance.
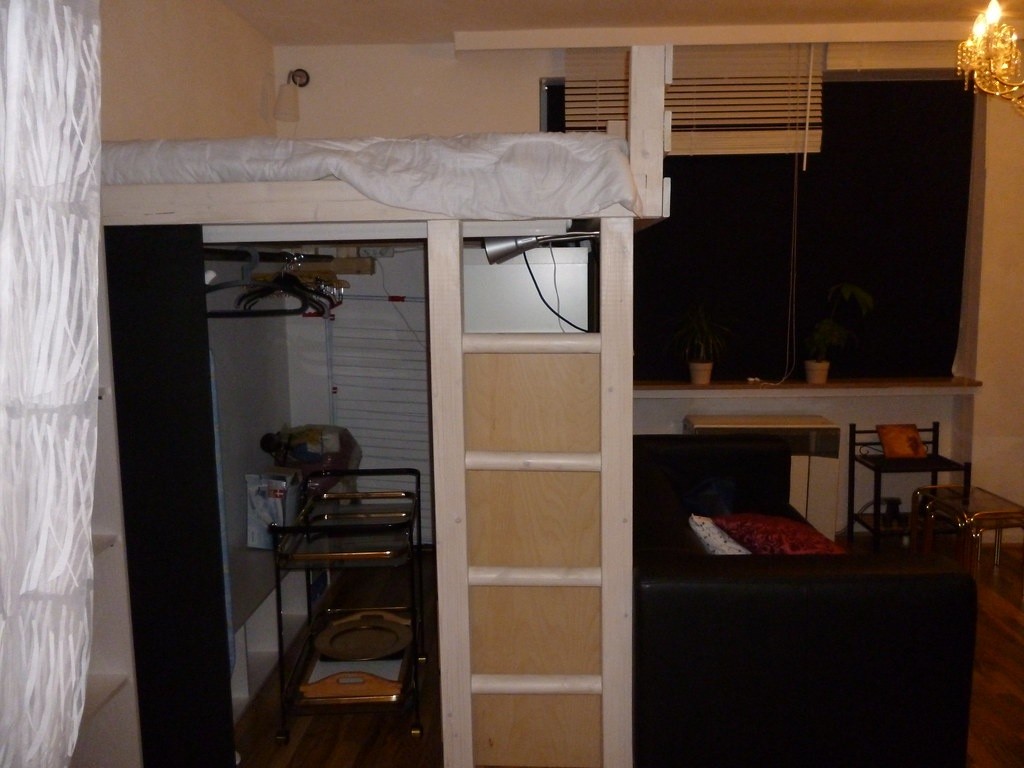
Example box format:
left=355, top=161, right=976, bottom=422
left=687, top=514, right=751, bottom=555
left=712, top=510, right=847, bottom=559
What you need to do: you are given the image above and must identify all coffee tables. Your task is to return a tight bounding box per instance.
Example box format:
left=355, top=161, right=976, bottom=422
left=911, top=483, right=1023, bottom=570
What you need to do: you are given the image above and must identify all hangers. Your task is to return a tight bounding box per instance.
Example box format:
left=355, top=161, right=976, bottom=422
left=205, top=245, right=352, bottom=318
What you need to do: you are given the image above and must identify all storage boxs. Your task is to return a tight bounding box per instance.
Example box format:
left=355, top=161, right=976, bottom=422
left=246, top=459, right=297, bottom=550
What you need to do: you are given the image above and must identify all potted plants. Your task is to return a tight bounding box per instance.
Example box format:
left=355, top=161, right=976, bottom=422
left=681, top=310, right=714, bottom=384
left=803, top=319, right=845, bottom=384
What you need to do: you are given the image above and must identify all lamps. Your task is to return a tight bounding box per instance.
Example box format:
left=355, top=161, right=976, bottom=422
left=954, top=0, right=1024, bottom=107
left=482, top=231, right=598, bottom=265
left=273, top=67, right=310, bottom=123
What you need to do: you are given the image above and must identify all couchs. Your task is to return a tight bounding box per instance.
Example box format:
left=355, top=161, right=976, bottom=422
left=633, top=435, right=977, bottom=767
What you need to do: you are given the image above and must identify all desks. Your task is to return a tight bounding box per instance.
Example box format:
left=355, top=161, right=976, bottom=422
left=683, top=411, right=839, bottom=521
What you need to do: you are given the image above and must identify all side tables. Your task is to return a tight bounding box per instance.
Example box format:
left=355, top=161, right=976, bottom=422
left=847, top=421, right=973, bottom=572
